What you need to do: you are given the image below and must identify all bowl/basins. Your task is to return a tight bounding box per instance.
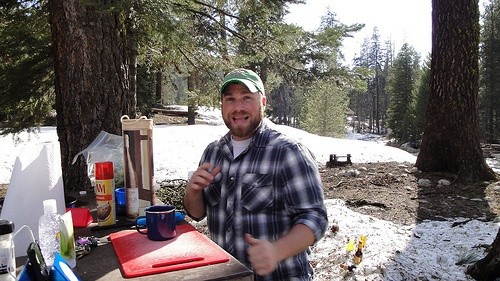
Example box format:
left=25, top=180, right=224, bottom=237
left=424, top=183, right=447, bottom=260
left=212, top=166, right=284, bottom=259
left=65, top=196, right=77, bottom=208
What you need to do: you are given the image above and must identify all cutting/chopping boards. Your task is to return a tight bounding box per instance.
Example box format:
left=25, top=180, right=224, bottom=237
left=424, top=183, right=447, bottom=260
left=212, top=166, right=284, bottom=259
left=109, top=224, right=231, bottom=279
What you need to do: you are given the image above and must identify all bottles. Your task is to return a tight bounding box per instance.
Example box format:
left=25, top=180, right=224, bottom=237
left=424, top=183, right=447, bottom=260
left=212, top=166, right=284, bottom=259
left=353, top=241, right=363, bottom=264
left=95, top=162, right=116, bottom=226
left=0, top=219, right=17, bottom=281
left=38, top=199, right=61, bottom=266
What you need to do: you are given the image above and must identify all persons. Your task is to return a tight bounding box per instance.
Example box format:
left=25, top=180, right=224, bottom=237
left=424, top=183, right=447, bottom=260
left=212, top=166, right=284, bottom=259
left=183, top=68, right=328, bottom=281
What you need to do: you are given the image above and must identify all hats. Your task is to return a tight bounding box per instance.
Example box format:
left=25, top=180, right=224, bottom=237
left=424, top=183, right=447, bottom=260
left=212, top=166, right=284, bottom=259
left=221, top=68, right=265, bottom=96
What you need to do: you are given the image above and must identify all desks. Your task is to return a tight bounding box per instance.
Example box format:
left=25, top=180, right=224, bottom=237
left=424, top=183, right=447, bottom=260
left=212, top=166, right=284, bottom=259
left=74, top=195, right=255, bottom=281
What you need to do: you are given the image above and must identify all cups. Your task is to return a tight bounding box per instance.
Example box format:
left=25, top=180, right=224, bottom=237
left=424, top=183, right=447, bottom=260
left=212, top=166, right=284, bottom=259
left=120, top=114, right=155, bottom=221
left=135, top=205, right=176, bottom=240
left=115, top=187, right=126, bottom=206
